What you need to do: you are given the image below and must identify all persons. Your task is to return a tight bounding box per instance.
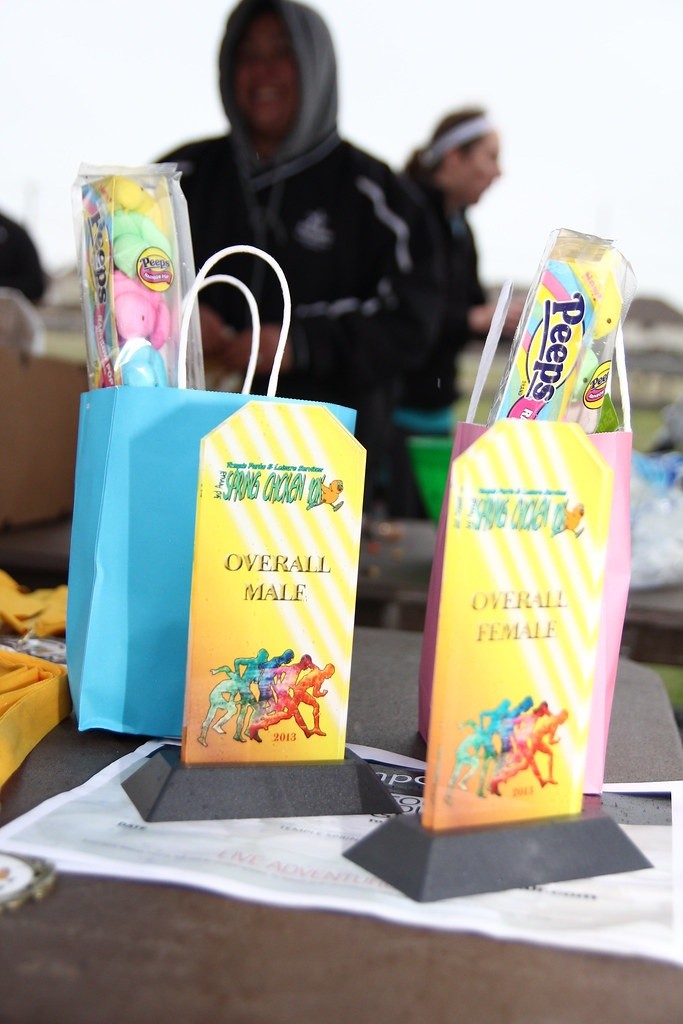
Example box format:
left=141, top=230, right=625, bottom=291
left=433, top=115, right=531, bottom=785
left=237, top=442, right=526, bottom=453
left=395, top=108, right=527, bottom=415
left=158, top=0, right=435, bottom=405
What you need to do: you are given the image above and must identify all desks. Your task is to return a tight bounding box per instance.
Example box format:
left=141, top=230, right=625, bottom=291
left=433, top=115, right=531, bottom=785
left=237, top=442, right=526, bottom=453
left=360, top=518, right=683, bottom=641
left=0, top=629, right=683, bottom=1024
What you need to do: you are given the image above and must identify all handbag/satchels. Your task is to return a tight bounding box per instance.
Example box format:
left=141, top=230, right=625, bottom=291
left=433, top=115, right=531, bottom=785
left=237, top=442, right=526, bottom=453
left=417, top=263, right=635, bottom=796
left=65, top=245, right=360, bottom=743
